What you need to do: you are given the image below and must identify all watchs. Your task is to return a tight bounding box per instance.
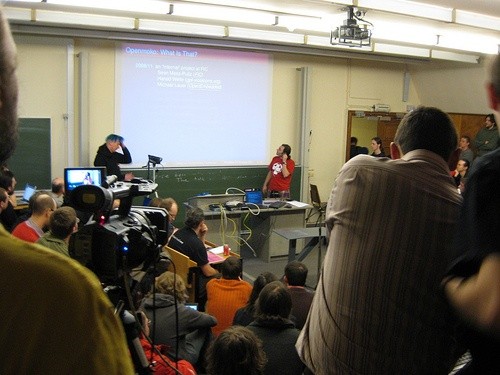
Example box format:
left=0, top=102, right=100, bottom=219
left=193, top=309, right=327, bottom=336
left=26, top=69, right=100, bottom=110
left=283, top=162, right=287, bottom=165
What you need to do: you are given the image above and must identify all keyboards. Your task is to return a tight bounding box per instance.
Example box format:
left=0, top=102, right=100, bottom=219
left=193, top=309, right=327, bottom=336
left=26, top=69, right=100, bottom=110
left=270, top=202, right=286, bottom=209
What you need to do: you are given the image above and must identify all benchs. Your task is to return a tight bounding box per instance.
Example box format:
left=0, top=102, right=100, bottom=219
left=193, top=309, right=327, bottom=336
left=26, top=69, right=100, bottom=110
left=165, top=246, right=198, bottom=302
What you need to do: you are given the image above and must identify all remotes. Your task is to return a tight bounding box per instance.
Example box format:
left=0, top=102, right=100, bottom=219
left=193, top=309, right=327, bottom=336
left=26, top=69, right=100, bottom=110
left=117, top=183, right=124, bottom=187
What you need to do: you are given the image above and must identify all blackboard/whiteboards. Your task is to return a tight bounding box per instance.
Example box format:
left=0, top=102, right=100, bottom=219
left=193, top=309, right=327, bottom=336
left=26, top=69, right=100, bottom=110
left=120, top=165, right=302, bottom=229
left=7, top=117, right=53, bottom=191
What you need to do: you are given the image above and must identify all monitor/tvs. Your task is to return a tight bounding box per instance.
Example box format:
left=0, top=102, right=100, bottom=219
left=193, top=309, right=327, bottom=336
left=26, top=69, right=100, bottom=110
left=64, top=167, right=106, bottom=190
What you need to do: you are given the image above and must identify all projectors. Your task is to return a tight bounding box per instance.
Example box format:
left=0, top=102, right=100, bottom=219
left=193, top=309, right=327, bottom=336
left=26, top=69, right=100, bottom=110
left=334, top=25, right=367, bottom=41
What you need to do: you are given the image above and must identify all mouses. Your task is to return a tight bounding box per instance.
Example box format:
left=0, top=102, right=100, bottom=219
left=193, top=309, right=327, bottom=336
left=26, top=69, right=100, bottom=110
left=285, top=206, right=292, bottom=208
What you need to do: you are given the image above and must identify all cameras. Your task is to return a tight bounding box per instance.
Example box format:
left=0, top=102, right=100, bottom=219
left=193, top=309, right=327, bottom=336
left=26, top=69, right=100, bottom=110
left=149, top=154, right=162, bottom=165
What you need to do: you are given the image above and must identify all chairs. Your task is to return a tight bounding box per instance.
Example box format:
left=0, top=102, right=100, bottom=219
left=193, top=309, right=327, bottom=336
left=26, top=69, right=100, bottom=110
left=305, top=184, right=329, bottom=226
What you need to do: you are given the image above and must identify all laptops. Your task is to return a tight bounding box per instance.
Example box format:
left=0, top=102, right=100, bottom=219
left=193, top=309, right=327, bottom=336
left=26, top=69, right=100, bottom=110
left=245, top=191, right=268, bottom=209
left=16, top=183, right=37, bottom=206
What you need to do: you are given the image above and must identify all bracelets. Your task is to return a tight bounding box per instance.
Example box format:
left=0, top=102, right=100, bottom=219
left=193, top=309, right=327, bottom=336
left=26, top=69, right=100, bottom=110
left=441, top=274, right=461, bottom=291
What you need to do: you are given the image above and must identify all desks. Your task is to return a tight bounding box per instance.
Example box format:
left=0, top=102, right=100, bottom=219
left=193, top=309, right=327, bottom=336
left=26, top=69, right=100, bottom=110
left=184, top=197, right=314, bottom=262
left=206, top=240, right=241, bottom=266
left=272, top=226, right=329, bottom=262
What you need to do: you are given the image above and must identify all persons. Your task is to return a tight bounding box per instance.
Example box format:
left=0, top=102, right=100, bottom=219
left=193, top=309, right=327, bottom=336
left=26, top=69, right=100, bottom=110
left=261, top=143, right=295, bottom=199
left=231, top=271, right=280, bottom=328
left=134, top=310, right=200, bottom=375
left=244, top=280, right=313, bottom=375
left=137, top=271, right=218, bottom=375
left=0, top=133, right=178, bottom=243
left=280, top=259, right=317, bottom=328
left=204, top=256, right=254, bottom=334
left=167, top=206, right=222, bottom=301
left=0, top=3, right=136, bottom=374
left=293, top=104, right=472, bottom=375
left=204, top=325, right=269, bottom=375
left=349, top=112, right=500, bottom=194
left=33, top=206, right=81, bottom=258
left=435, top=56, right=500, bottom=375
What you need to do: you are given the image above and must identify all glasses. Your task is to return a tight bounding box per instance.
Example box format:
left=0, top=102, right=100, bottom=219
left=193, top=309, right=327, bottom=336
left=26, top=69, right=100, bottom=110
left=142, top=319, right=152, bottom=330
left=76, top=218, right=80, bottom=224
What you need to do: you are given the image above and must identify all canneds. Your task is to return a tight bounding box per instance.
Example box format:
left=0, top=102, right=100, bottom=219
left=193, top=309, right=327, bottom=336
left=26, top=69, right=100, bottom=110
left=223, top=243, right=229, bottom=256
